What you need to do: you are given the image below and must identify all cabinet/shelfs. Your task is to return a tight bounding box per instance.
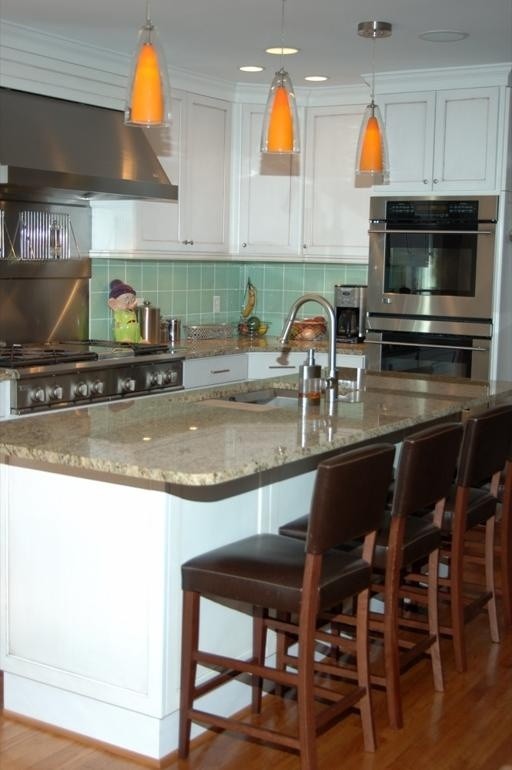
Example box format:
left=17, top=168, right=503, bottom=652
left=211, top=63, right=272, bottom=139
left=89, top=42, right=237, bottom=261
left=183, top=352, right=248, bottom=390
left=361, top=62, right=512, bottom=194
left=234, top=81, right=372, bottom=265
left=248, top=351, right=364, bottom=381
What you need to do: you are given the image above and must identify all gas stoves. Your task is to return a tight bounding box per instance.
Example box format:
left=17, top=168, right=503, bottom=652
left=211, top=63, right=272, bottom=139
left=0, top=337, right=186, bottom=416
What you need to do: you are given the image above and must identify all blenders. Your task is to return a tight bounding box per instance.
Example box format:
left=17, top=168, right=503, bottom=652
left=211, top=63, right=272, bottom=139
left=332, top=285, right=367, bottom=345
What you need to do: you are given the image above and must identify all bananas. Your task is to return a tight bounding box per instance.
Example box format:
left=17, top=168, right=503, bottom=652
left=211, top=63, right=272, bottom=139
left=242, top=286, right=256, bottom=317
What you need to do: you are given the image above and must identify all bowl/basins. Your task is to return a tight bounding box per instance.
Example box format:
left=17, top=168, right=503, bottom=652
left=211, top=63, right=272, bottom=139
left=236, top=321, right=272, bottom=337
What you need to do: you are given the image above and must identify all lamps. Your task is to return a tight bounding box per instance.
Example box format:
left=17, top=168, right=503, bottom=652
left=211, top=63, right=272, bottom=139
left=261, top=0, right=302, bottom=154
left=125, top=0, right=172, bottom=126
left=357, top=21, right=391, bottom=172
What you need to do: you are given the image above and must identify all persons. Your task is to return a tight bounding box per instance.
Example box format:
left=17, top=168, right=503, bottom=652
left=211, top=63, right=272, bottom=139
left=108, top=279, right=151, bottom=344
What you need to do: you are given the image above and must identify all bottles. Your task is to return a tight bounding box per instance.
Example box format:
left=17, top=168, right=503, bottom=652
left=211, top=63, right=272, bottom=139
left=136, top=302, right=162, bottom=345
left=160, top=317, right=181, bottom=343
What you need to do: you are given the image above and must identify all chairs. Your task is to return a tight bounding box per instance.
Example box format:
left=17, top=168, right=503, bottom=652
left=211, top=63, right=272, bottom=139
left=179, top=442, right=396, bottom=770
left=274, top=420, right=466, bottom=730
left=397, top=403, right=512, bottom=674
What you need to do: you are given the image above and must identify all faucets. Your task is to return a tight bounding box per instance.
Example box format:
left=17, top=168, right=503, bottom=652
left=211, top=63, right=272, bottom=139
left=278, top=291, right=337, bottom=396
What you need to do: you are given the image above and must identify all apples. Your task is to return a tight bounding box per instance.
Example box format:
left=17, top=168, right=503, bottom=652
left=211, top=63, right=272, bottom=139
left=238, top=316, right=267, bottom=335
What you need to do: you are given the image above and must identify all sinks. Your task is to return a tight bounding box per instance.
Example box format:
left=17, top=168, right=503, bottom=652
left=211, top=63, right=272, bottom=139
left=191, top=379, right=346, bottom=407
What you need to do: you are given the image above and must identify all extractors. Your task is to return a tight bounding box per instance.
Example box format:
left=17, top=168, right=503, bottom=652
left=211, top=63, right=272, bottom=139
left=0, top=85, right=178, bottom=207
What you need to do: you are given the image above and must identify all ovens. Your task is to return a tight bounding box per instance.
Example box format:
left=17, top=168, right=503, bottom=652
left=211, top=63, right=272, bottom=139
left=365, top=317, right=493, bottom=387
left=365, top=196, right=499, bottom=322
